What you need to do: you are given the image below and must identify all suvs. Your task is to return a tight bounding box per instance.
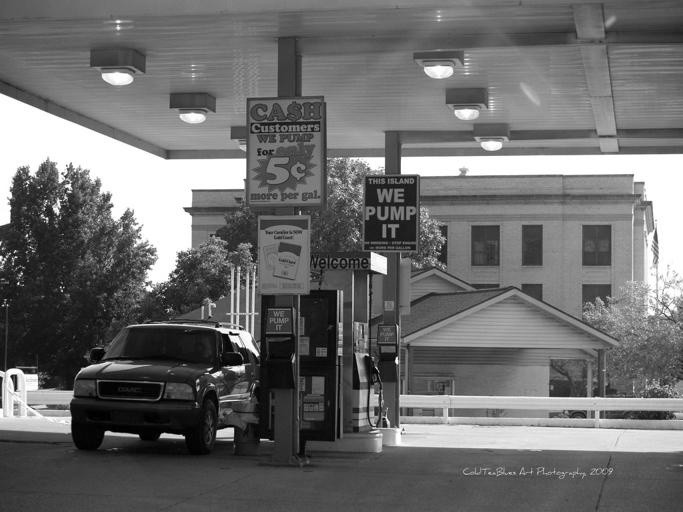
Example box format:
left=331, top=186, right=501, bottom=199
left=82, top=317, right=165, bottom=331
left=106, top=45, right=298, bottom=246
left=68, top=320, right=261, bottom=456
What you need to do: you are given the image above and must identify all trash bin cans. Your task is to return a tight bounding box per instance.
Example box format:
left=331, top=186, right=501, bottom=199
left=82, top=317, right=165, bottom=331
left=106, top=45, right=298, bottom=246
left=262, top=351, right=296, bottom=389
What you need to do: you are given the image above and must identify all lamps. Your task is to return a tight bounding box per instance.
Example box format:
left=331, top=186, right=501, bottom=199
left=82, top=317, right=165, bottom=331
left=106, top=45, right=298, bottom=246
left=473, top=123, right=512, bottom=152
left=414, top=51, right=465, bottom=80
left=231, top=126, right=247, bottom=152
left=90, top=47, right=146, bottom=86
left=445, top=88, right=488, bottom=121
left=170, top=93, right=216, bottom=124
